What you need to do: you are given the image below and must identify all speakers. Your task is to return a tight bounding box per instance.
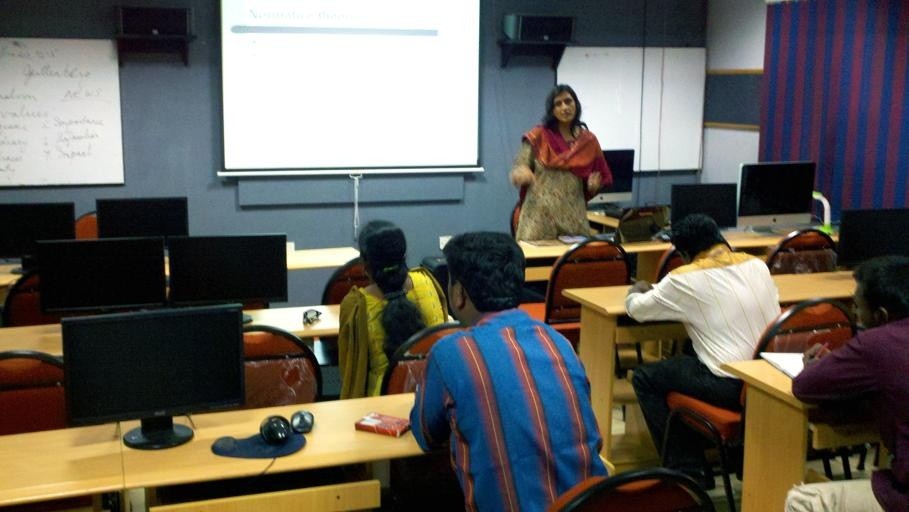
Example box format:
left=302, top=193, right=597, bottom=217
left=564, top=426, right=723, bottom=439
left=116, top=2, right=195, bottom=38
left=503, top=13, right=574, bottom=43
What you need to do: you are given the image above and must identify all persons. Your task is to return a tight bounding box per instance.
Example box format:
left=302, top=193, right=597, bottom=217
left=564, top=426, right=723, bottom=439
left=407, top=229, right=609, bottom=512
left=621, top=208, right=787, bottom=490
left=506, top=82, right=615, bottom=243
left=780, top=249, right=908, bottom=512
left=334, top=218, right=448, bottom=403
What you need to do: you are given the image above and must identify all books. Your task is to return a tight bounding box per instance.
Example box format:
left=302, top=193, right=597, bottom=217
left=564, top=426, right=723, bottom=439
left=758, top=349, right=806, bottom=380
left=352, top=410, right=412, bottom=439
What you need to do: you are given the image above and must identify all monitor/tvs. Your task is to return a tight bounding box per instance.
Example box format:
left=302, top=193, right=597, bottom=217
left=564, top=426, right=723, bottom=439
left=166, top=234, right=288, bottom=323
left=95, top=197, right=189, bottom=248
left=587, top=150, right=634, bottom=204
left=836, top=207, right=909, bottom=266
left=671, top=183, right=736, bottom=237
left=62, top=303, right=245, bottom=449
left=0, top=202, right=75, bottom=273
left=736, top=161, right=816, bottom=237
left=36, top=236, right=166, bottom=316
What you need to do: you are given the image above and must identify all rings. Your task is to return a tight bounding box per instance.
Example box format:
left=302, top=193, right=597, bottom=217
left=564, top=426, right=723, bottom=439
left=588, top=181, right=593, bottom=187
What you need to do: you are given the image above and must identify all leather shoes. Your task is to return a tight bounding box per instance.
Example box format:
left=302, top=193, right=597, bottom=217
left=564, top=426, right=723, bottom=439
left=664, top=462, right=715, bottom=490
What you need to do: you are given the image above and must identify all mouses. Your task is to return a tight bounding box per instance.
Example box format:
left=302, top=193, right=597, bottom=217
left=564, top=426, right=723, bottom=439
left=260, top=416, right=290, bottom=446
left=291, top=411, right=314, bottom=433
left=659, top=232, right=671, bottom=241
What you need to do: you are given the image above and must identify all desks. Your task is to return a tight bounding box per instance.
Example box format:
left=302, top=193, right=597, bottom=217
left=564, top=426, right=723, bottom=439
left=1, top=419, right=124, bottom=511
left=2, top=246, right=361, bottom=284
left=118, top=388, right=426, bottom=509
left=440, top=226, right=840, bottom=322
left=1, top=301, right=460, bottom=360
left=717, top=355, right=883, bottom=509
left=560, top=270, right=861, bottom=463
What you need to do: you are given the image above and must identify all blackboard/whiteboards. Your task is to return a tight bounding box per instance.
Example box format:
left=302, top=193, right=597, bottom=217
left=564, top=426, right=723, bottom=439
left=0, top=38, right=127, bottom=185
left=697, top=124, right=761, bottom=186
left=556, top=47, right=707, bottom=174
left=706, top=3, right=768, bottom=77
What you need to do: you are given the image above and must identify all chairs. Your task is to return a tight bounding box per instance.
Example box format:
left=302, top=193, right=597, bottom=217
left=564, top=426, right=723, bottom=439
left=763, top=225, right=842, bottom=274
left=656, top=296, right=858, bottom=510
left=515, top=234, right=630, bottom=421
left=511, top=201, right=522, bottom=239
left=545, top=467, right=713, bottom=510
left=1, top=348, right=64, bottom=438
left=653, top=246, right=687, bottom=286
left=75, top=210, right=99, bottom=240
left=241, top=324, right=323, bottom=411
